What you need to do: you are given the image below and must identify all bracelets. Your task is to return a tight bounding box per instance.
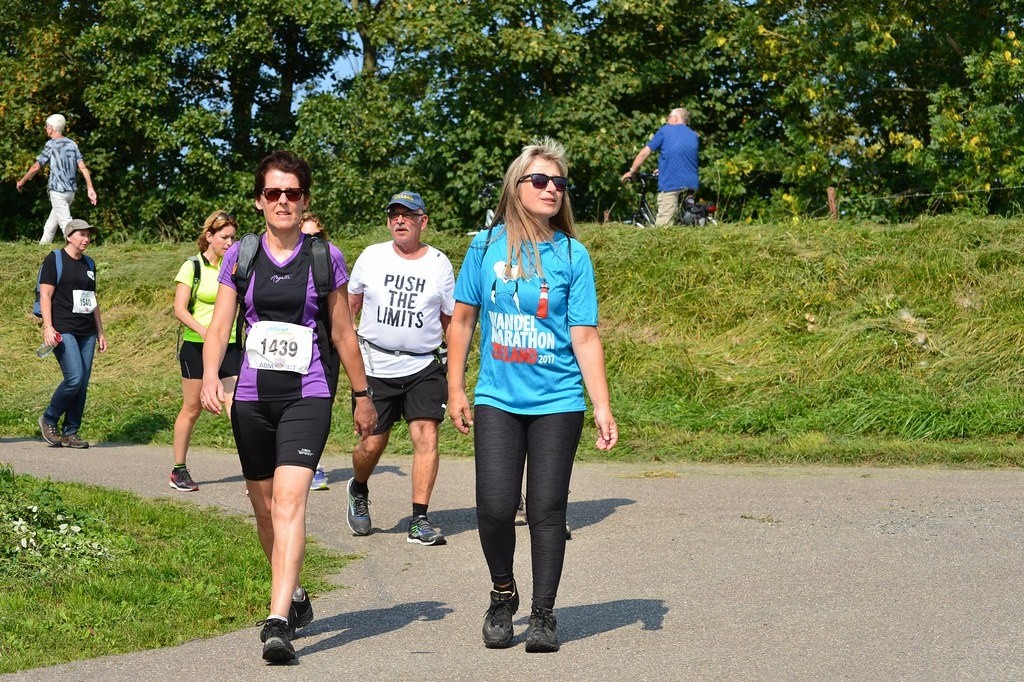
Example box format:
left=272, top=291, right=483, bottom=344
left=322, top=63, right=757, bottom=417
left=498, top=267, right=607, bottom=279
left=630, top=168, right=637, bottom=174
left=44, top=324, right=52, bottom=330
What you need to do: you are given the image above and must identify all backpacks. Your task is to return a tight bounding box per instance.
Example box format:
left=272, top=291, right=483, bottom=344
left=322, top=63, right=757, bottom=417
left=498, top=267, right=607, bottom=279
left=34, top=249, right=95, bottom=317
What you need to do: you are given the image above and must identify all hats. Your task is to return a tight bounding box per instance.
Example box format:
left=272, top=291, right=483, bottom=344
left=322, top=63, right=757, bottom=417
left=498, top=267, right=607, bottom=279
left=386, top=191, right=426, bottom=215
left=65, top=219, right=99, bottom=242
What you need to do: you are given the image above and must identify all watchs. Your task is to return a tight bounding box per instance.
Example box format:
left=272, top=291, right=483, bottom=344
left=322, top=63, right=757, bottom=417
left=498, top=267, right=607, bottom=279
left=355, top=386, right=374, bottom=398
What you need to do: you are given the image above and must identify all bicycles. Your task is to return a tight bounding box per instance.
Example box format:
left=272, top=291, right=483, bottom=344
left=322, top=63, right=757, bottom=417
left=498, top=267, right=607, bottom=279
left=621, top=172, right=719, bottom=230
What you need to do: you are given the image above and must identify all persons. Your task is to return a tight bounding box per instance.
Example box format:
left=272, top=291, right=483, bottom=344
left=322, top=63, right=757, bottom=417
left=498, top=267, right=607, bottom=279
left=38, top=219, right=107, bottom=449
left=345, top=190, right=469, bottom=545
left=202, top=151, right=379, bottom=661
left=447, top=135, right=617, bottom=653
left=168, top=211, right=251, bottom=495
left=16, top=114, right=97, bottom=244
left=621, top=108, right=699, bottom=231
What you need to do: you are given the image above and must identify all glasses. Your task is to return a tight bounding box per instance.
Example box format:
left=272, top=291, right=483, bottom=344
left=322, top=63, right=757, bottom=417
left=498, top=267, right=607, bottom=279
left=305, top=232, right=325, bottom=239
left=262, top=188, right=305, bottom=202
left=518, top=173, right=568, bottom=191
left=387, top=211, right=423, bottom=221
left=210, top=213, right=237, bottom=229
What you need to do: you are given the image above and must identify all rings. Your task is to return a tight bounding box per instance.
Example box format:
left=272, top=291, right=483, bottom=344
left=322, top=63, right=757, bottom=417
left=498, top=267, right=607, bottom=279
left=373, top=424, right=377, bottom=428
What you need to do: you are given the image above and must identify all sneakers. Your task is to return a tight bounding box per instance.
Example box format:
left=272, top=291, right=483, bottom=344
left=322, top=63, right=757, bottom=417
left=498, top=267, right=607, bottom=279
left=347, top=477, right=372, bottom=535
left=566, top=524, right=572, bottom=540
left=169, top=467, right=198, bottom=492
left=39, top=416, right=61, bottom=446
left=61, top=433, right=89, bottom=448
left=288, top=585, right=314, bottom=640
left=310, top=468, right=329, bottom=490
left=257, top=617, right=296, bottom=664
left=525, top=602, right=558, bottom=651
left=514, top=494, right=526, bottom=526
left=482, top=578, right=519, bottom=648
left=407, top=515, right=444, bottom=545
